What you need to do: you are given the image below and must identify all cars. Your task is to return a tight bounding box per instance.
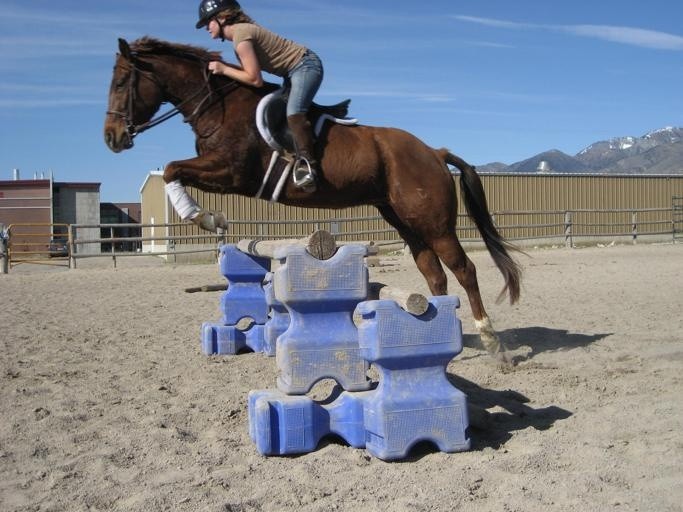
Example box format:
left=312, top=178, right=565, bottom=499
left=49, top=240, right=67, bottom=257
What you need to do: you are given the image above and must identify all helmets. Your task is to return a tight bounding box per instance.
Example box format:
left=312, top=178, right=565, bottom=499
left=194, top=0, right=238, bottom=29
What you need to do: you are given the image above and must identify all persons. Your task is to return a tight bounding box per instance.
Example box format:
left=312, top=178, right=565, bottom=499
left=196, top=1, right=324, bottom=189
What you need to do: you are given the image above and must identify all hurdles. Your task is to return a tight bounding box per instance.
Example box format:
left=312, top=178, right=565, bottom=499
left=200, top=231, right=471, bottom=460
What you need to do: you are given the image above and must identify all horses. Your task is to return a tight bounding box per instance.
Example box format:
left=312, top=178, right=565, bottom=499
left=103, top=35, right=524, bottom=359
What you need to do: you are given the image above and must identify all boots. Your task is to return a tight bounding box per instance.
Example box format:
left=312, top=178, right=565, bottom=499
left=286, top=111, right=317, bottom=183
